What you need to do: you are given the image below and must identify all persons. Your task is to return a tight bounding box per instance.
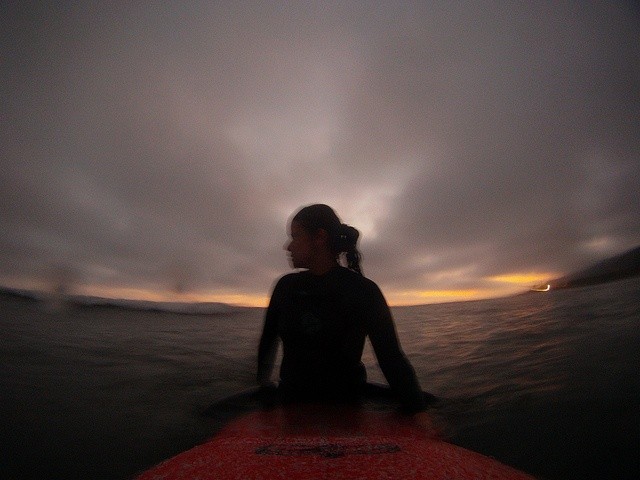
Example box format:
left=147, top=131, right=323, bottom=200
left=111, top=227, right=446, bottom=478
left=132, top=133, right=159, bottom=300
left=256, top=204, right=425, bottom=412
